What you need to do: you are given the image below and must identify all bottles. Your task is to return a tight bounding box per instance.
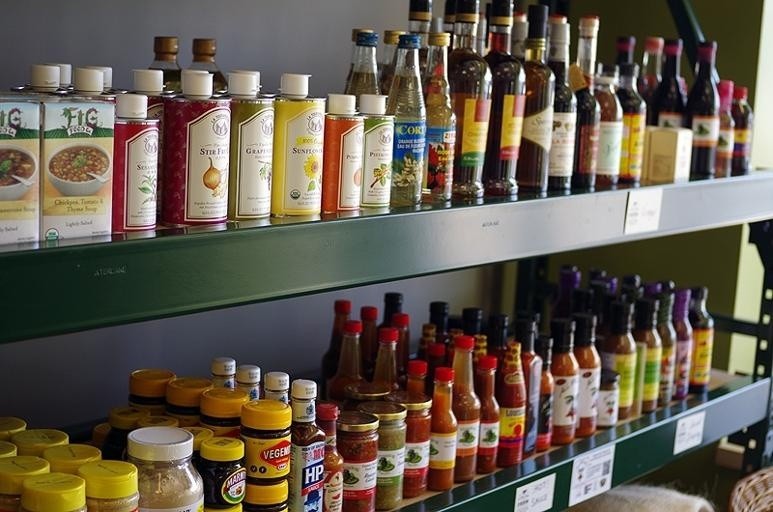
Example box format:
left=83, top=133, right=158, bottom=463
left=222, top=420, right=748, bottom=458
left=0, top=264, right=713, bottom=512
left=10, top=0, right=753, bottom=244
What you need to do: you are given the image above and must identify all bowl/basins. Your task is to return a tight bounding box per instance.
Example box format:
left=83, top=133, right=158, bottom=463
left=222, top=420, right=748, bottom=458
left=1, top=144, right=37, bottom=201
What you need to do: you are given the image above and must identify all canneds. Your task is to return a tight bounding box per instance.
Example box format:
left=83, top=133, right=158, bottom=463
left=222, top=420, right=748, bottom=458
left=334, top=381, right=433, bottom=512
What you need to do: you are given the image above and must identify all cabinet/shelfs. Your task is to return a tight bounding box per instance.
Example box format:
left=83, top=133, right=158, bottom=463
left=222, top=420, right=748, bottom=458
left=1, top=168, right=773, bottom=511
